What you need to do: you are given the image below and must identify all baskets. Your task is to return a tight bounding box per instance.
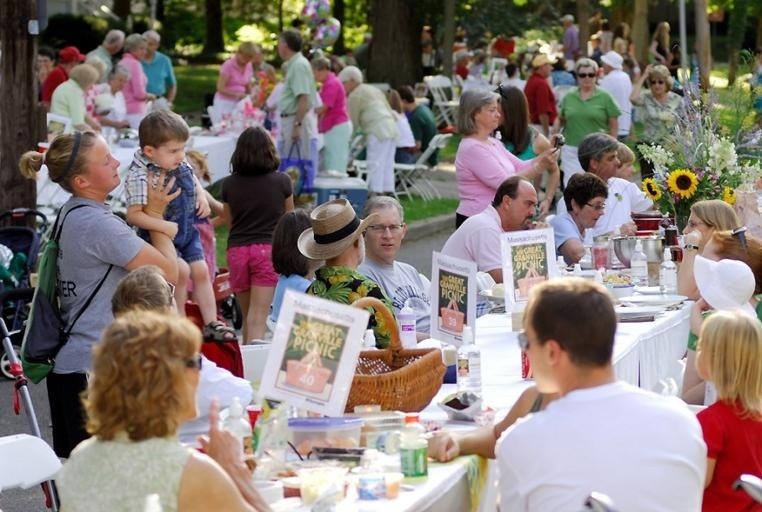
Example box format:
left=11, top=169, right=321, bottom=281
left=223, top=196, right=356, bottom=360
left=344, top=298, right=445, bottom=412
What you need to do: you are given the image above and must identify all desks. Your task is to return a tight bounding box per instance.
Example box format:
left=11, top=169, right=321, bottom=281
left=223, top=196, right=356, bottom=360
left=33, top=118, right=296, bottom=225
left=190, top=228, right=710, bottom=512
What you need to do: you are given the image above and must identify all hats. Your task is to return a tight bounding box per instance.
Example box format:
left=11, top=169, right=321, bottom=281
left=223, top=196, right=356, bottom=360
left=691, top=252, right=756, bottom=323
left=530, top=14, right=623, bottom=69
left=298, top=197, right=380, bottom=260
left=60, top=48, right=85, bottom=63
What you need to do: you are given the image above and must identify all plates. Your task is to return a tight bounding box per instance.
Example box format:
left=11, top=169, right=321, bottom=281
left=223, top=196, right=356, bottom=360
left=568, top=268, right=619, bottom=278
left=616, top=294, right=690, bottom=306
left=612, top=307, right=666, bottom=319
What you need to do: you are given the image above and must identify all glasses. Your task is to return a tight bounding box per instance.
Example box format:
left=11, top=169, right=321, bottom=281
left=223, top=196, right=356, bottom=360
left=578, top=73, right=595, bottom=78
left=651, top=80, right=664, bottom=85
left=368, top=225, right=403, bottom=234
left=496, top=83, right=506, bottom=100
left=164, top=284, right=203, bottom=375
left=586, top=203, right=606, bottom=209
left=686, top=219, right=705, bottom=227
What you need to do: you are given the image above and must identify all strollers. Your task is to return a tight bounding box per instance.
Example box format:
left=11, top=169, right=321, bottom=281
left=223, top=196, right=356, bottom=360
left=184, top=300, right=245, bottom=384
left=0, top=206, right=49, bottom=382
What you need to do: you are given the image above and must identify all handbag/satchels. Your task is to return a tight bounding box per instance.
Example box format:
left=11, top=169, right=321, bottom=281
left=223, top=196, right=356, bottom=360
left=279, top=139, right=313, bottom=202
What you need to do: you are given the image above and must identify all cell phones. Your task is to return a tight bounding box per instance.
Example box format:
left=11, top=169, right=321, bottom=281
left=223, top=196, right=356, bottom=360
left=312, top=446, right=366, bottom=460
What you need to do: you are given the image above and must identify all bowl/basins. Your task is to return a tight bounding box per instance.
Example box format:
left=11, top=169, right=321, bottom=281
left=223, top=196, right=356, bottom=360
left=611, top=236, right=666, bottom=268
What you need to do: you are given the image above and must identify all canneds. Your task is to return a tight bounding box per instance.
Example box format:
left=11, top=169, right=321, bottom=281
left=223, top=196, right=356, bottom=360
left=521, top=351, right=534, bottom=379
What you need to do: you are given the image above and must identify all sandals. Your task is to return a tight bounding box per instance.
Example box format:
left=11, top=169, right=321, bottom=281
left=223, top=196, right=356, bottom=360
left=204, top=321, right=238, bottom=344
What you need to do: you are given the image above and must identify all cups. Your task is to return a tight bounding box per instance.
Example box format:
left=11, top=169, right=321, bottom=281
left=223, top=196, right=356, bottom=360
left=592, top=243, right=610, bottom=270
left=211, top=104, right=265, bottom=140
left=248, top=461, right=405, bottom=512
left=244, top=404, right=262, bottom=429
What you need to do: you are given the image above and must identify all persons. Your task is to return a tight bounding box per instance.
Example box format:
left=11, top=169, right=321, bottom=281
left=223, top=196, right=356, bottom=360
left=19, top=14, right=762, bottom=355
left=695, top=311, right=761, bottom=512
left=57, top=306, right=272, bottom=512
left=482, top=277, right=708, bottom=512
left=111, top=264, right=254, bottom=448
left=428, top=385, right=562, bottom=462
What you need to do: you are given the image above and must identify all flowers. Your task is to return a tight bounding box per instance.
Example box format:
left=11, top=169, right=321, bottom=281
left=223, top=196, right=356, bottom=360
left=623, top=63, right=746, bottom=223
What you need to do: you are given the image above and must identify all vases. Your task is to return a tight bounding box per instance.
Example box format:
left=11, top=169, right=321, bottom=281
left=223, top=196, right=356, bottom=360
left=666, top=207, right=697, bottom=243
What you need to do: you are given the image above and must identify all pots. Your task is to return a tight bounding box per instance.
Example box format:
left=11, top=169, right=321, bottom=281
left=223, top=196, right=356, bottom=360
left=630, top=211, right=663, bottom=230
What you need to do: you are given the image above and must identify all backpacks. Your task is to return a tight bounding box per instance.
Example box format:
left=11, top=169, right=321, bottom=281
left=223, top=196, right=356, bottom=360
left=20, top=200, right=112, bottom=385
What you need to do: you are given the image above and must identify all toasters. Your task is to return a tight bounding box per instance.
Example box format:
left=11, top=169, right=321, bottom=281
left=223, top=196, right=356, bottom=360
left=629, top=239, right=649, bottom=288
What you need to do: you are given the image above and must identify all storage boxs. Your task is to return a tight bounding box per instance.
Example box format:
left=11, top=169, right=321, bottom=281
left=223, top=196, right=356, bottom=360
left=305, top=173, right=367, bottom=214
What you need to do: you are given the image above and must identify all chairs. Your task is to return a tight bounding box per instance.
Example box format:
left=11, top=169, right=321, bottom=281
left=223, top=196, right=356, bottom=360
left=423, top=74, right=461, bottom=128
left=0, top=430, right=64, bottom=510
left=355, top=130, right=451, bottom=204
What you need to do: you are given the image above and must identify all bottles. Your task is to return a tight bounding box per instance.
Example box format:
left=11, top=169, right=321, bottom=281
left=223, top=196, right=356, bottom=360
left=456, top=326, right=483, bottom=391
left=221, top=395, right=253, bottom=466
left=657, top=247, right=679, bottom=296
left=398, top=414, right=429, bottom=485
left=664, top=229, right=678, bottom=261
left=395, top=301, right=418, bottom=350
left=556, top=255, right=604, bottom=283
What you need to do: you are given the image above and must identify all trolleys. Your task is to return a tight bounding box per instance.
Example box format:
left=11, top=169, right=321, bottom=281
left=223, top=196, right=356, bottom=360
left=0, top=316, right=70, bottom=512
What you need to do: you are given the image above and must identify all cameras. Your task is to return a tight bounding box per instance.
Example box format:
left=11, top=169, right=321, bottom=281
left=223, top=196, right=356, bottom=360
left=554, top=136, right=565, bottom=151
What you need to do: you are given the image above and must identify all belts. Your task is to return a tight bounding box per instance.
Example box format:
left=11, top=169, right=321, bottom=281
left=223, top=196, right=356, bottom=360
left=279, top=112, right=289, bottom=116
